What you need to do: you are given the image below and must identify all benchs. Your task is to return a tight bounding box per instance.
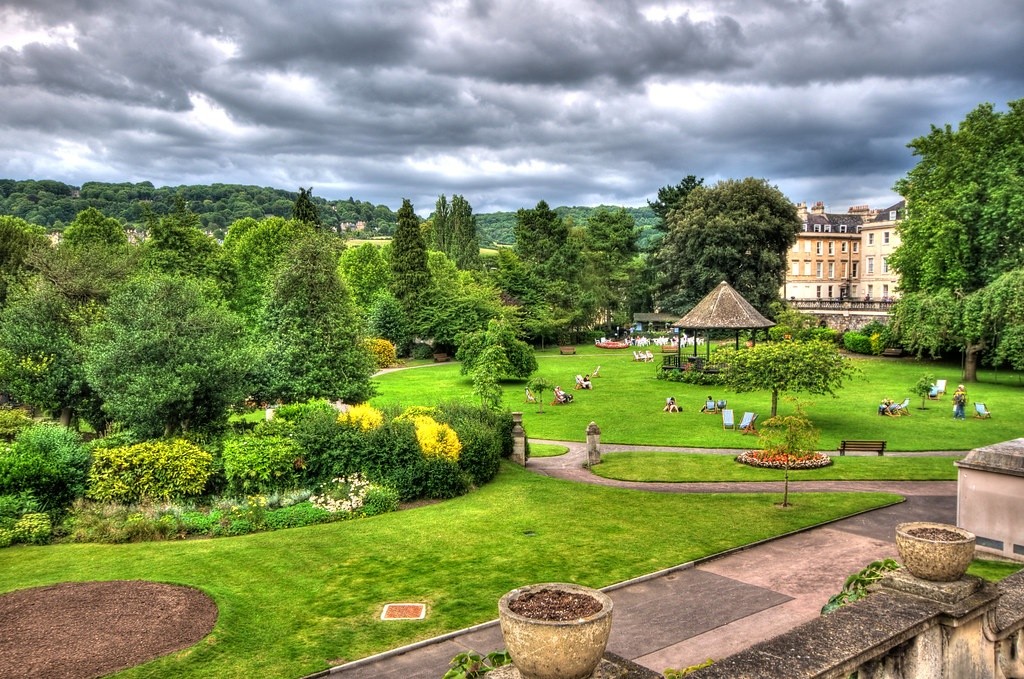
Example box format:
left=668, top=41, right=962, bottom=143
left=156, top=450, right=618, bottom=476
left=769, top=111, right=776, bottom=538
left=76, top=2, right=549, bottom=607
left=837, top=440, right=887, bottom=456
left=433, top=353, right=450, bottom=363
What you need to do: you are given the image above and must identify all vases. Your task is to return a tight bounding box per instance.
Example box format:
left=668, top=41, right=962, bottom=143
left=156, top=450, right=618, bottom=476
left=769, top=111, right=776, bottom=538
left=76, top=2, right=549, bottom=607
left=496, top=582, right=614, bottom=679
left=894, top=521, right=976, bottom=581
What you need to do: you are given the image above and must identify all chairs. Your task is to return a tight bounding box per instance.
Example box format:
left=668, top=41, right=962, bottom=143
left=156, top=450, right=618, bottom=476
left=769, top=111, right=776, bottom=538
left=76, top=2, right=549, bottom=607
left=556, top=334, right=996, bottom=436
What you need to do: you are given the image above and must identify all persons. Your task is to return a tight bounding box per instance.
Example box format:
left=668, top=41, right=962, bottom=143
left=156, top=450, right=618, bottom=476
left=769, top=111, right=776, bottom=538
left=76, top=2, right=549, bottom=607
left=625, top=335, right=641, bottom=346
left=663, top=396, right=676, bottom=412
left=671, top=332, right=688, bottom=349
left=699, top=395, right=713, bottom=413
left=878, top=399, right=895, bottom=417
left=525, top=387, right=537, bottom=403
left=952, top=384, right=967, bottom=421
left=575, top=374, right=592, bottom=390
left=555, top=386, right=572, bottom=403
